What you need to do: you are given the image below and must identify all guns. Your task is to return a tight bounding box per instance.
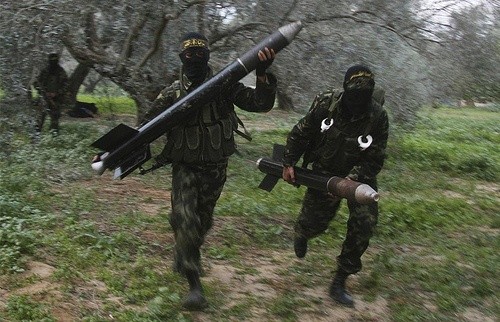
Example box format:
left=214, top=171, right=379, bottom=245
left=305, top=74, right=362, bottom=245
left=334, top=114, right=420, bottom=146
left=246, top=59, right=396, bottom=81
left=139, top=159, right=169, bottom=175
left=296, top=160, right=309, bottom=188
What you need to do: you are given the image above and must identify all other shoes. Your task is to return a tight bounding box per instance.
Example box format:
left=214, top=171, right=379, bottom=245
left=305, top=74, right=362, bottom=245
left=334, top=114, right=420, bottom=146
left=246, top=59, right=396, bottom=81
left=181, top=271, right=210, bottom=313
left=292, top=234, right=307, bottom=259
left=329, top=268, right=355, bottom=308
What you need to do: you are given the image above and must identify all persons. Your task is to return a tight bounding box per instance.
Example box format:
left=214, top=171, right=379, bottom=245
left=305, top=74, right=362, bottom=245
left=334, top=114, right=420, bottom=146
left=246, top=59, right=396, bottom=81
left=92, top=31, right=277, bottom=310
left=282, top=66, right=389, bottom=306
left=35, top=53, right=67, bottom=136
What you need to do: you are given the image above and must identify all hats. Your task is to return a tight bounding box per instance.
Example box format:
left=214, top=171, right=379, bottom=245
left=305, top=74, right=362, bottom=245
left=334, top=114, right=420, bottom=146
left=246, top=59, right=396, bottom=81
left=343, top=65, right=375, bottom=87
left=177, top=31, right=211, bottom=56
